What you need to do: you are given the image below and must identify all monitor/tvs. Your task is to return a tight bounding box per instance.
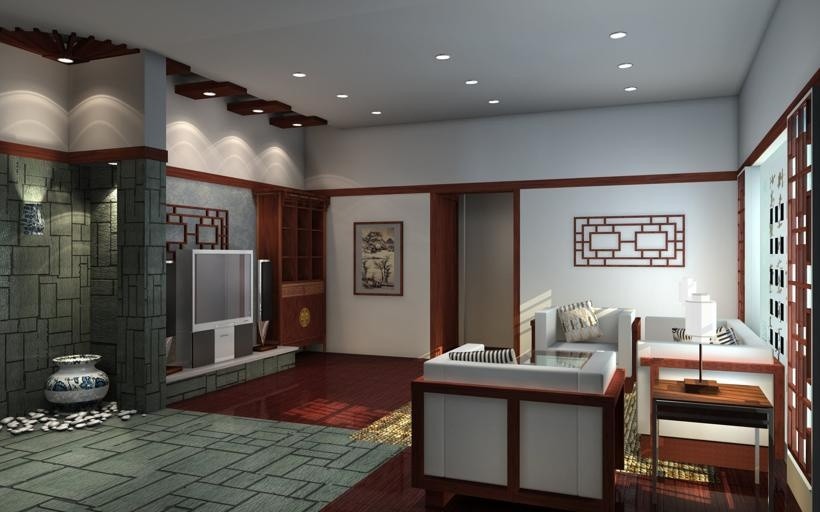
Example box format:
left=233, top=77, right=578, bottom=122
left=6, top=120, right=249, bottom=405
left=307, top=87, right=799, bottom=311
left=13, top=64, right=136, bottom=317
left=192, top=249, right=254, bottom=333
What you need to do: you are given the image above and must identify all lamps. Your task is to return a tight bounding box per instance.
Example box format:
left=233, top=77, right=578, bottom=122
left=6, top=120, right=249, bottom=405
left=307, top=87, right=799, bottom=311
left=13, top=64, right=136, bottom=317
left=20, top=199, right=46, bottom=238
left=684, top=291, right=721, bottom=396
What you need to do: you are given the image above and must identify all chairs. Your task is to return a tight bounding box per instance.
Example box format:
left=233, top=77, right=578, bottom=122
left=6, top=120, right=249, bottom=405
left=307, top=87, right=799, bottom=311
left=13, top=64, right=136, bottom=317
left=530, top=307, right=642, bottom=394
left=407, top=342, right=628, bottom=512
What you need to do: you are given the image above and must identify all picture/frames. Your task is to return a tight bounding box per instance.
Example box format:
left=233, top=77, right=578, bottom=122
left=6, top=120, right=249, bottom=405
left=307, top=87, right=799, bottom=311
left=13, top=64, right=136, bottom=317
left=352, top=219, right=405, bottom=297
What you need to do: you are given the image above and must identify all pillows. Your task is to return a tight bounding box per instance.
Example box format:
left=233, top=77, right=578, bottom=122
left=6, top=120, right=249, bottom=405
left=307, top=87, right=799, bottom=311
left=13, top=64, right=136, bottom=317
left=670, top=324, right=742, bottom=347
left=449, top=348, right=517, bottom=364
left=556, top=299, right=600, bottom=342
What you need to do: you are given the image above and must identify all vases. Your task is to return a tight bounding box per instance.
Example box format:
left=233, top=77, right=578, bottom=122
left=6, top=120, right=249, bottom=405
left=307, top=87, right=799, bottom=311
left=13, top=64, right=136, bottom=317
left=44, top=353, right=112, bottom=408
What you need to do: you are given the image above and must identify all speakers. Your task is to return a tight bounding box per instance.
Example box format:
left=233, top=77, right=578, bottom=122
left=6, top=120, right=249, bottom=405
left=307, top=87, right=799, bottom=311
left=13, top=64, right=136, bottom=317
left=165, top=258, right=178, bottom=338
left=258, top=259, right=273, bottom=320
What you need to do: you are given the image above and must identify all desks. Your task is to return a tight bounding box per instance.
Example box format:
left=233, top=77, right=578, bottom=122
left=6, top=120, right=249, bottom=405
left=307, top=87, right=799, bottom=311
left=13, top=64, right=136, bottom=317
left=650, top=379, right=778, bottom=512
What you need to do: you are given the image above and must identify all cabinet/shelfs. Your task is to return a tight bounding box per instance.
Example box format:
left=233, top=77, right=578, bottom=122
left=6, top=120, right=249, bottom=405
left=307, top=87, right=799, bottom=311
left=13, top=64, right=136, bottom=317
left=166, top=346, right=299, bottom=405
left=252, top=186, right=328, bottom=362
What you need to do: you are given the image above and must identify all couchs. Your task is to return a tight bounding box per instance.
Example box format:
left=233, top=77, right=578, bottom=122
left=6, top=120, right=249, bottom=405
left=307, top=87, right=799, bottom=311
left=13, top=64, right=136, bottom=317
left=638, top=316, right=787, bottom=494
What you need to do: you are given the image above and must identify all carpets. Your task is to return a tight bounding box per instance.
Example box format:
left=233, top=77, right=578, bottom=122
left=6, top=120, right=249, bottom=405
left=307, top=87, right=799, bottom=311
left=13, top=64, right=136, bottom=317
left=350, top=381, right=718, bottom=485
left=0, top=407, right=406, bottom=512
